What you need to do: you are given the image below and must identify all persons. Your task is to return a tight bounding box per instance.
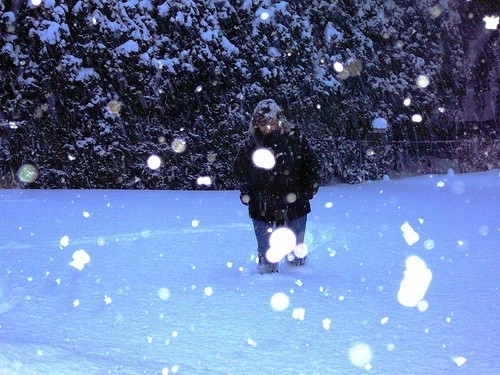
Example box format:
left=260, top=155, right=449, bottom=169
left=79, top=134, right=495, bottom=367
left=233, top=99, right=323, bottom=273
